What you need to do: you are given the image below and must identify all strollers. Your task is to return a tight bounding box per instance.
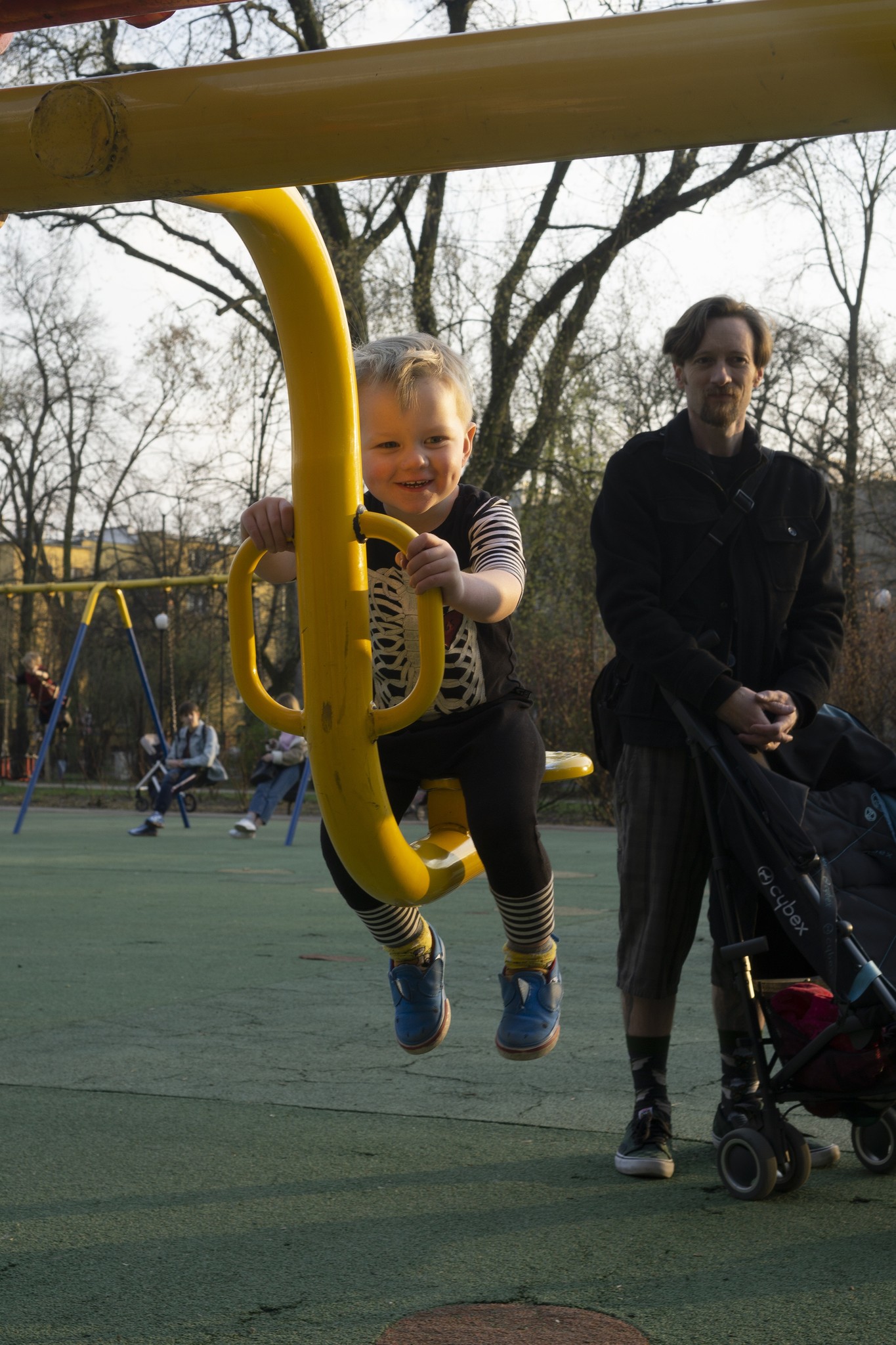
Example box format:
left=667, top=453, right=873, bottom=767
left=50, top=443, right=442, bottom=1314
left=649, top=683, right=896, bottom=1200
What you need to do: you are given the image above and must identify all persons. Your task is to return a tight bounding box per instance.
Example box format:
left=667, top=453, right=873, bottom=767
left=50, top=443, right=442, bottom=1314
left=229, top=693, right=309, bottom=840
left=5, top=651, right=72, bottom=730
left=589, top=297, right=846, bottom=1178
left=128, top=701, right=217, bottom=837
left=240, top=333, right=563, bottom=1063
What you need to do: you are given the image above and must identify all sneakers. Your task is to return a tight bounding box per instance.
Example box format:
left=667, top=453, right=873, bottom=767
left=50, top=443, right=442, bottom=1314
left=388, top=925, right=451, bottom=1053
left=128, top=824, right=157, bottom=835
left=711, top=1105, right=841, bottom=1169
left=146, top=810, right=165, bottom=828
left=616, top=1104, right=675, bottom=1176
left=228, top=829, right=254, bottom=839
left=235, top=818, right=257, bottom=832
left=496, top=935, right=562, bottom=1060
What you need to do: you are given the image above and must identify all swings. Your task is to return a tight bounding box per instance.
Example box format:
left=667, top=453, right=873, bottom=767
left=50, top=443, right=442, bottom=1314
left=254, top=588, right=306, bottom=803
left=163, top=589, right=216, bottom=788
left=9, top=597, right=71, bottom=711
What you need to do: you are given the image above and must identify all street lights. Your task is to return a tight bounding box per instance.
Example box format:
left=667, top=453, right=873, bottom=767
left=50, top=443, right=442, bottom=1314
left=156, top=612, right=167, bottom=729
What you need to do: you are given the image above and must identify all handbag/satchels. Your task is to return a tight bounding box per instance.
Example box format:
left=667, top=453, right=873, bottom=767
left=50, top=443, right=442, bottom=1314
left=592, top=653, right=637, bottom=769
left=249, top=761, right=282, bottom=785
left=772, top=981, right=881, bottom=1087
